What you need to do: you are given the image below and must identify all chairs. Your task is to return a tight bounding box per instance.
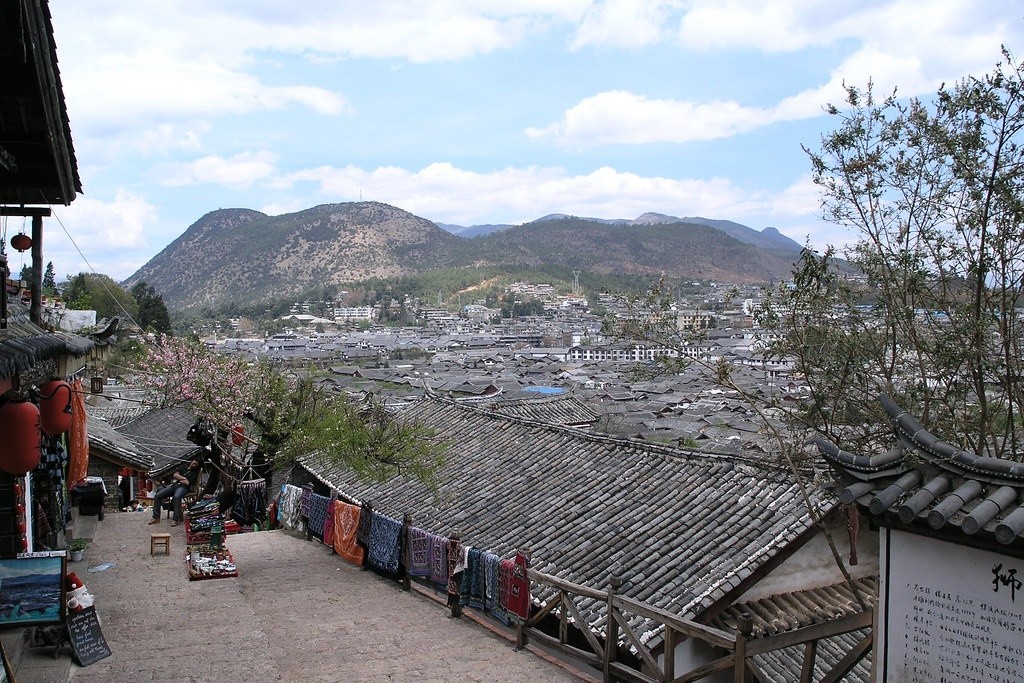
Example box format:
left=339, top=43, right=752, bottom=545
left=153, top=468, right=203, bottom=519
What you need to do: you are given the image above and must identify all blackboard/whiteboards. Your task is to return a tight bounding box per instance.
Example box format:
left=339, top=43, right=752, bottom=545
left=65, top=605, right=111, bottom=667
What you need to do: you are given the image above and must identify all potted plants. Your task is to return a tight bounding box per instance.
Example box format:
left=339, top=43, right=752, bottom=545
left=67, top=539, right=86, bottom=562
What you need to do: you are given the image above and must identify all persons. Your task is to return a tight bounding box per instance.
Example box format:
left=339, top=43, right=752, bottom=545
left=148, top=456, right=204, bottom=527
left=130, top=499, right=144, bottom=512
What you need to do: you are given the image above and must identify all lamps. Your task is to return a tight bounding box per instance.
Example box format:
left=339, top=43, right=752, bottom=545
left=91, top=361, right=103, bottom=393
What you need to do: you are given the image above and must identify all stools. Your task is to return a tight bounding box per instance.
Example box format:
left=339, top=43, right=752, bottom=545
left=151, top=533, right=171, bottom=556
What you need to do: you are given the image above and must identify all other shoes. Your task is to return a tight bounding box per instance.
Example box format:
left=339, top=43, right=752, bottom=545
left=148, top=519, right=160, bottom=525
left=171, top=520, right=177, bottom=526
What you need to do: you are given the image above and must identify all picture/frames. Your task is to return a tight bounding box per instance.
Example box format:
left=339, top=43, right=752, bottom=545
left=0, top=550, right=67, bottom=630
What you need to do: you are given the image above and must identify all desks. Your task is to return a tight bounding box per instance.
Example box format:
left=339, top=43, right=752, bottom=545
left=135, top=496, right=154, bottom=507
left=224, top=522, right=239, bottom=536
